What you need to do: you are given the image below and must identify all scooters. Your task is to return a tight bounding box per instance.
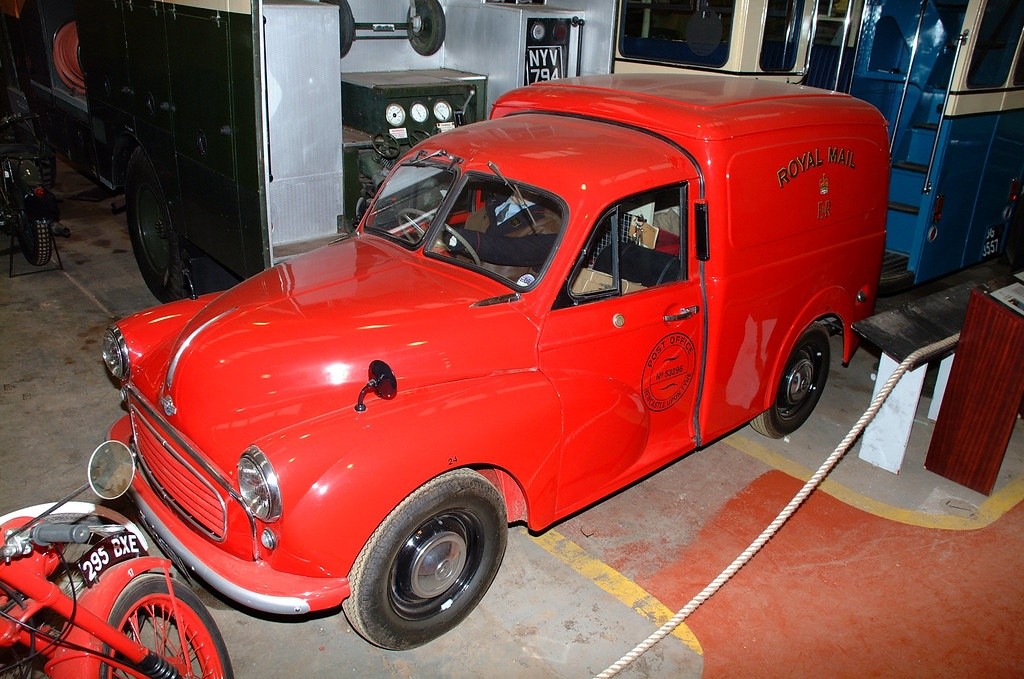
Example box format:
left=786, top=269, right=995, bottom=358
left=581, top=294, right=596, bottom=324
left=0, top=107, right=64, bottom=278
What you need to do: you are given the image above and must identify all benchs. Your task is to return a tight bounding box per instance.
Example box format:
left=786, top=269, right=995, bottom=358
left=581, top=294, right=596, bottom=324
left=622, top=36, right=856, bottom=93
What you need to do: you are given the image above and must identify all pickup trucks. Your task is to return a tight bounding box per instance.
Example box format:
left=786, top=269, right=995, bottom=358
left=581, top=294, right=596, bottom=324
left=103, top=73, right=892, bottom=654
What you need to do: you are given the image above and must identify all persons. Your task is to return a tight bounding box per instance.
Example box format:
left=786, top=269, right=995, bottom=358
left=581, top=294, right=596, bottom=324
left=442, top=182, right=642, bottom=310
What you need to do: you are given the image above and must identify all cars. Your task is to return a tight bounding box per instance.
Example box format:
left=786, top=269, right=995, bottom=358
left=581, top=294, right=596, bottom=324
left=1, top=0, right=616, bottom=304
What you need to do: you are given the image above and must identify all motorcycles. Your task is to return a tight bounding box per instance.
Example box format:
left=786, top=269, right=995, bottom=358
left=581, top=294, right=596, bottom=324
left=0, top=439, right=237, bottom=679
